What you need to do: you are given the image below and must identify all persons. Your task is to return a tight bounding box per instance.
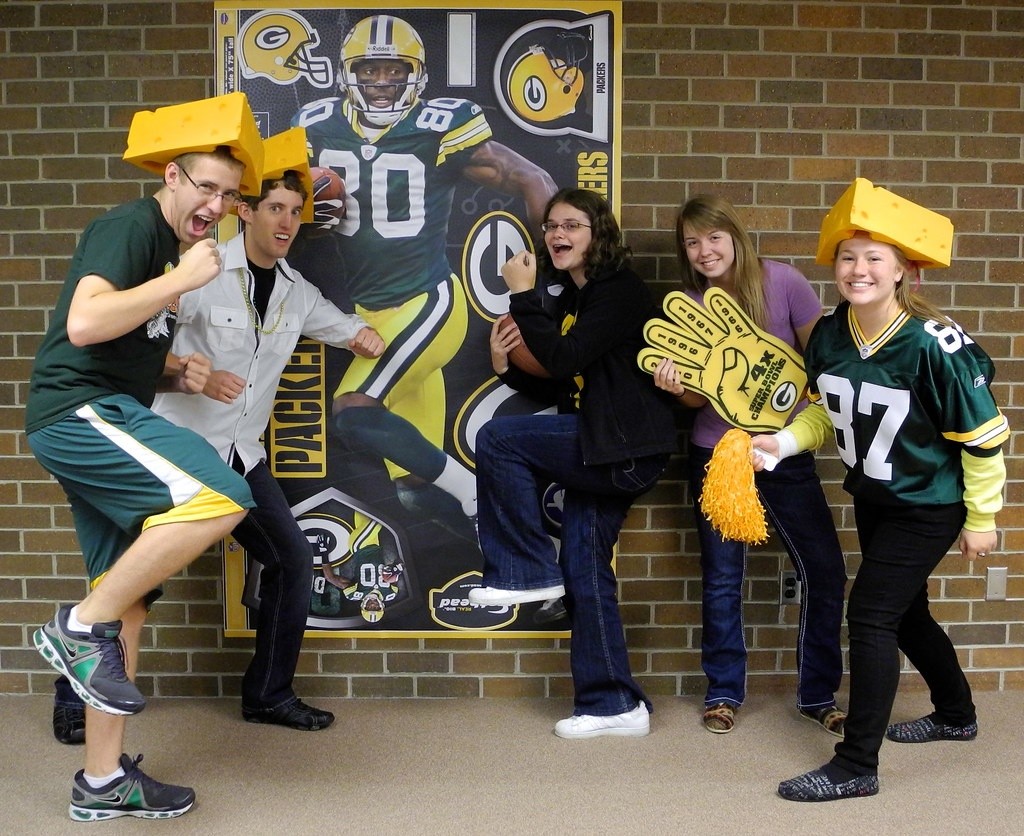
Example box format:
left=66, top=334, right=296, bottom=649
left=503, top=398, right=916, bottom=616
left=24, top=92, right=265, bottom=822
left=317, top=510, right=402, bottom=622
left=284, top=15, right=565, bottom=622
left=747, top=178, right=1011, bottom=802
left=52, top=126, right=386, bottom=746
left=654, top=194, right=851, bottom=738
left=469, top=187, right=675, bottom=739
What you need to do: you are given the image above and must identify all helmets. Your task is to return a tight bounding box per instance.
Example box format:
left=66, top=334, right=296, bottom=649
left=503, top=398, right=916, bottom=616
left=340, top=15, right=428, bottom=126
left=360, top=593, right=383, bottom=623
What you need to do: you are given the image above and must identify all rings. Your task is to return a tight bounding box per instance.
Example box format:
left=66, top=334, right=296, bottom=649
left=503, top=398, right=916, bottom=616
left=978, top=552, right=985, bottom=557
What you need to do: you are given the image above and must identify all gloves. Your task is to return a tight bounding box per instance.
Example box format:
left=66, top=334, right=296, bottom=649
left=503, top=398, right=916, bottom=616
left=303, top=177, right=344, bottom=231
left=316, top=534, right=330, bottom=552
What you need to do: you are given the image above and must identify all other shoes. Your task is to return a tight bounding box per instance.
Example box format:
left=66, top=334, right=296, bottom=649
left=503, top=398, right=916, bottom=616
left=533, top=599, right=569, bottom=623
left=468, top=512, right=484, bottom=556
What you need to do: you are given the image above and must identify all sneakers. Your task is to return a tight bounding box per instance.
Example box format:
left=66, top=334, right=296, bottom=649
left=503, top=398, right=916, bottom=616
left=778, top=763, right=880, bottom=801
left=69, top=753, right=196, bottom=822
left=703, top=703, right=739, bottom=733
left=242, top=697, right=335, bottom=731
left=33, top=605, right=147, bottom=715
left=799, top=703, right=848, bottom=738
left=53, top=674, right=85, bottom=743
left=555, top=700, right=651, bottom=740
left=469, top=585, right=566, bottom=606
left=886, top=714, right=978, bottom=743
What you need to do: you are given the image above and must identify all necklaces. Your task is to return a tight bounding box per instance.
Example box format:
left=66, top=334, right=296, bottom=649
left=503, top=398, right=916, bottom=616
left=238, top=268, right=283, bottom=335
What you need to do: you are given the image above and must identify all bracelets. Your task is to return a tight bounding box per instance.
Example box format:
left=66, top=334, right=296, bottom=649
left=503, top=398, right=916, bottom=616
left=677, top=388, right=686, bottom=398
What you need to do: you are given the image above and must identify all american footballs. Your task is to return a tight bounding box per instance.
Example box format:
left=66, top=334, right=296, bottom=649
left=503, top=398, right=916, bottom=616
left=298, top=166, right=347, bottom=241
left=498, top=314, right=551, bottom=381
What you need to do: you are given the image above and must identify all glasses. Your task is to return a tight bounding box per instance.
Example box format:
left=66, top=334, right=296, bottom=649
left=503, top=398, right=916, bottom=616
left=540, top=221, right=595, bottom=234
left=176, top=162, right=242, bottom=208
